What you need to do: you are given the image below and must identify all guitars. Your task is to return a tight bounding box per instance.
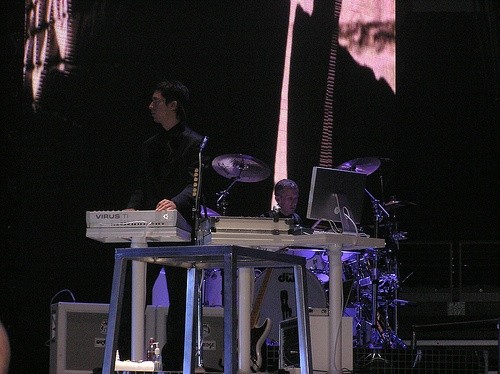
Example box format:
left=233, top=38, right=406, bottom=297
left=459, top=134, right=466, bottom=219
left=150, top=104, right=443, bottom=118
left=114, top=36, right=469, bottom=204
left=223, top=268, right=273, bottom=374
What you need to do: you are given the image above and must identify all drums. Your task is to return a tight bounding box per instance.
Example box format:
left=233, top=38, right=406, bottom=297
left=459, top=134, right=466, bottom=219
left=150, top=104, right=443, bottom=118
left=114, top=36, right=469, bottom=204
left=320, top=249, right=373, bottom=284
left=305, top=255, right=329, bottom=284
left=152, top=263, right=256, bottom=308
left=250, top=264, right=328, bottom=348
left=285, top=249, right=324, bottom=271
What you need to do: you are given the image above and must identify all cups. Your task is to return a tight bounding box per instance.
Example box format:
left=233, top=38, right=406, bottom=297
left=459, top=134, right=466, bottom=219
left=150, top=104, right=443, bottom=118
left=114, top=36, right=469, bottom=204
left=147, top=337, right=157, bottom=361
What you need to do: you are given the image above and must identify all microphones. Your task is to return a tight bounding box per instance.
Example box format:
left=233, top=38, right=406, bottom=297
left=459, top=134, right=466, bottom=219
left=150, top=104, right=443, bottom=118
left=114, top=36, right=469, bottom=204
left=199, top=135, right=209, bottom=152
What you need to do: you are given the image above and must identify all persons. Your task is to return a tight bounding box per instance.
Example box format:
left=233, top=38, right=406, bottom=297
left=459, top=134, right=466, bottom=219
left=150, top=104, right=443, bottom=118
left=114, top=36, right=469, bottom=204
left=92, top=81, right=207, bottom=374
left=254, top=179, right=303, bottom=278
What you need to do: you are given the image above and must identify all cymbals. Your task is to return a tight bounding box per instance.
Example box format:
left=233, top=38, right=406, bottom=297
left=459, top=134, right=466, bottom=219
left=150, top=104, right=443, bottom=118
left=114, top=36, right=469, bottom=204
left=385, top=200, right=417, bottom=209
left=335, top=156, right=381, bottom=176
left=210, top=153, right=272, bottom=183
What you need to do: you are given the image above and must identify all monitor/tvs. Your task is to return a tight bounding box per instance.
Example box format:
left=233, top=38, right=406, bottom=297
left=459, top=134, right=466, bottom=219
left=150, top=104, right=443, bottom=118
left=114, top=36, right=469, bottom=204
left=306, top=166, right=366, bottom=236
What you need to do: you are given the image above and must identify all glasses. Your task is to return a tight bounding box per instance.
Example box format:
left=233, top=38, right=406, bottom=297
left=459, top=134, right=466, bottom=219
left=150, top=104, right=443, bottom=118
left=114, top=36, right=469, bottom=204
left=151, top=97, right=165, bottom=106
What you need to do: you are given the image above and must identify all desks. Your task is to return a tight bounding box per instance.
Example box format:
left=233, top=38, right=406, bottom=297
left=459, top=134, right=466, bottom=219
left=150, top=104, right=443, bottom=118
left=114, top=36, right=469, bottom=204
left=101, top=246, right=312, bottom=374
